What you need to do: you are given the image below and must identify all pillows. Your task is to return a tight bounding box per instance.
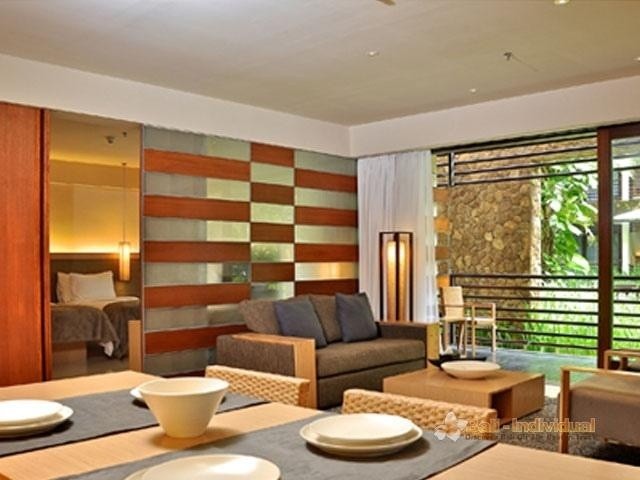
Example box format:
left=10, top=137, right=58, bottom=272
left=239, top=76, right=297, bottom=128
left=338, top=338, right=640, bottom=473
left=239, top=292, right=379, bottom=348
left=56, top=270, right=69, bottom=304
left=69, top=269, right=115, bottom=306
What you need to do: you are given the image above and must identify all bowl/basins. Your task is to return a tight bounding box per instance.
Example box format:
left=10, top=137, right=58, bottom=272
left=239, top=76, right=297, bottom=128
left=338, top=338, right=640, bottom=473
left=426, top=354, right=501, bottom=380
left=140, top=376, right=228, bottom=438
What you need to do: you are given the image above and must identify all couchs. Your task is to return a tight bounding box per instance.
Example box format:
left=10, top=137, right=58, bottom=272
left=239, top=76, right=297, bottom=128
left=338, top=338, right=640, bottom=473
left=216, top=321, right=440, bottom=410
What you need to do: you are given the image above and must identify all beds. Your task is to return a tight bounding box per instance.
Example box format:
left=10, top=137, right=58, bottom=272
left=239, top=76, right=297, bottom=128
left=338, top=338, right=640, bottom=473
left=50, top=296, right=141, bottom=378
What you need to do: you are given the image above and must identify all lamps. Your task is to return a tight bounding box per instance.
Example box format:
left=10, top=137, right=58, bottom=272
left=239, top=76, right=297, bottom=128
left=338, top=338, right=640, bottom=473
left=379, top=231, right=413, bottom=323
left=119, top=162, right=131, bottom=283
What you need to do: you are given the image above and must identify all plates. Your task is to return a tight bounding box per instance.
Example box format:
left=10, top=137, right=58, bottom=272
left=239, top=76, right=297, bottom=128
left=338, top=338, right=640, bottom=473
left=0, top=407, right=73, bottom=440
left=301, top=420, right=420, bottom=459
left=0, top=398, right=60, bottom=425
left=123, top=454, right=281, bottom=480
left=131, top=385, right=145, bottom=408
left=312, top=413, right=412, bottom=444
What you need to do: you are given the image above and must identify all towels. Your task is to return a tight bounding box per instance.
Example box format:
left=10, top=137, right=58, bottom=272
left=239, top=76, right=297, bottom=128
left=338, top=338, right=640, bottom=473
left=0, top=385, right=271, bottom=458
left=48, top=413, right=499, bottom=480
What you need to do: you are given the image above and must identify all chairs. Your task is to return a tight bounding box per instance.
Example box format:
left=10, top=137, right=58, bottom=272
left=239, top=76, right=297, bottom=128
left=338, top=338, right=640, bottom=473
left=205, top=365, right=312, bottom=408
left=440, top=286, right=496, bottom=362
left=341, top=389, right=499, bottom=441
left=558, top=348, right=640, bottom=454
